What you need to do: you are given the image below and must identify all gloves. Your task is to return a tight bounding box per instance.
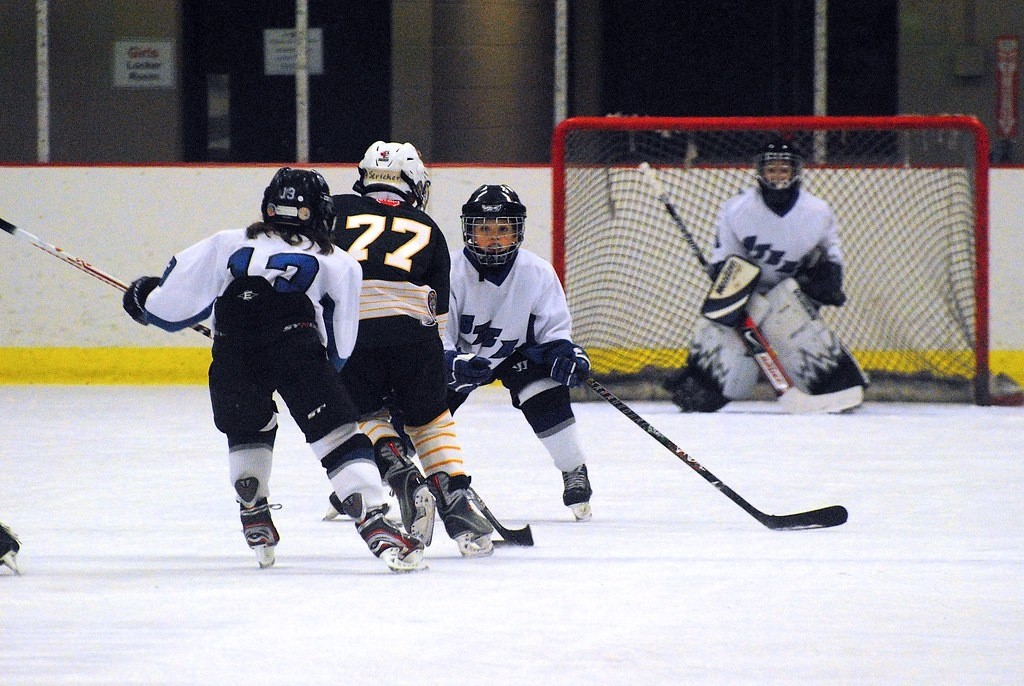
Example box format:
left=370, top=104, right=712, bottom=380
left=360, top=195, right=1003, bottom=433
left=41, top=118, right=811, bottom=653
left=522, top=339, right=591, bottom=389
left=794, top=262, right=848, bottom=309
left=444, top=349, right=493, bottom=394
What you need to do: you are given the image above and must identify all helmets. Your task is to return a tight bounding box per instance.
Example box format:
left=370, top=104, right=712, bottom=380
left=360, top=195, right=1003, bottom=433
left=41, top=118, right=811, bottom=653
left=352, top=140, right=431, bottom=204
left=461, top=184, right=527, bottom=245
left=754, top=140, right=804, bottom=217
left=259, top=166, right=337, bottom=239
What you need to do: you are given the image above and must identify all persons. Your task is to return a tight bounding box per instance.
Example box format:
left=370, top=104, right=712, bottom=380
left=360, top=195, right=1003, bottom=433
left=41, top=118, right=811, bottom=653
left=122, top=166, right=429, bottom=572
left=332, top=140, right=495, bottom=559
left=321, top=184, right=593, bottom=521
left=664, top=140, right=873, bottom=414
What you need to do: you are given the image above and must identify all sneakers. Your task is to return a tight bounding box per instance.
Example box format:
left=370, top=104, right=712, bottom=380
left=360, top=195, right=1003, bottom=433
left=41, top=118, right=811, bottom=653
left=425, top=470, right=495, bottom=558
left=234, top=477, right=280, bottom=569
left=381, top=439, right=436, bottom=548
left=341, top=492, right=429, bottom=572
left=562, top=463, right=594, bottom=522
left=0, top=523, right=23, bottom=576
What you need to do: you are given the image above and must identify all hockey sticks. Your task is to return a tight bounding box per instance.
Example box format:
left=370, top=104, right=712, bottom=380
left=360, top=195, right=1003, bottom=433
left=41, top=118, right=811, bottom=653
left=1, top=215, right=218, bottom=340
left=465, top=483, right=536, bottom=549
left=637, top=158, right=866, bottom=415
left=580, top=370, right=849, bottom=534
